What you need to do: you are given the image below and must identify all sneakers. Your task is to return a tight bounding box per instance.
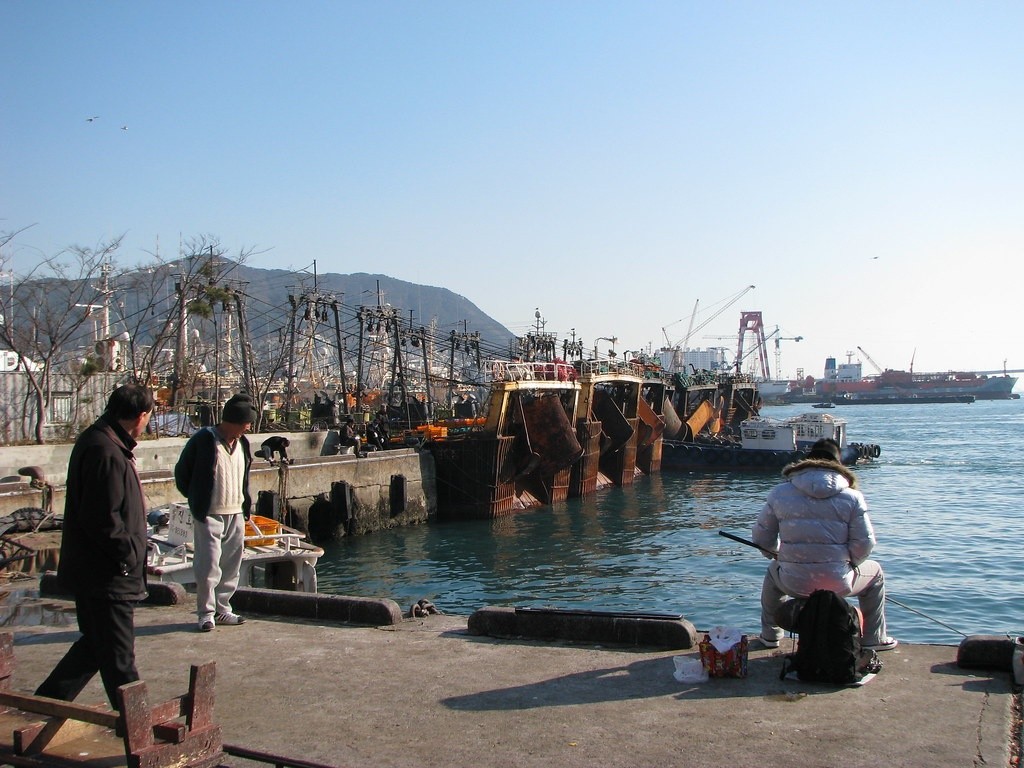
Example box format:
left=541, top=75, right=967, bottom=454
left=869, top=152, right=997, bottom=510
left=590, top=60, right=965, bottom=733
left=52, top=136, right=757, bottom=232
left=862, top=637, right=898, bottom=650
left=198, top=615, right=215, bottom=630
left=215, top=611, right=246, bottom=624
left=759, top=633, right=780, bottom=647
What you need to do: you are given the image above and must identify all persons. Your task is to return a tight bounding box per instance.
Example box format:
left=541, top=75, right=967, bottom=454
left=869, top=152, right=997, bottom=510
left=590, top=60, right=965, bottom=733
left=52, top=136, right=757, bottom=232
left=367, top=404, right=389, bottom=451
left=340, top=419, right=364, bottom=458
left=175, top=389, right=257, bottom=630
left=261, top=436, right=290, bottom=460
left=752, top=436, right=897, bottom=650
left=34, top=384, right=154, bottom=712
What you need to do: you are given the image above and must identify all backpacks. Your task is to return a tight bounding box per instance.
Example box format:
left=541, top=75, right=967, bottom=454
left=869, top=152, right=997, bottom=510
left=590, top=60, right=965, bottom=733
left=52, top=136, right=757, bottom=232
left=794, top=588, right=863, bottom=683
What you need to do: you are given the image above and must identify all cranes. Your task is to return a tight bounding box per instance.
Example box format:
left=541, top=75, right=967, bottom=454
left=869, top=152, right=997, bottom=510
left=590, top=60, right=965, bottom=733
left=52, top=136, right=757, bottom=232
left=660, top=285, right=755, bottom=373
left=704, top=325, right=804, bottom=384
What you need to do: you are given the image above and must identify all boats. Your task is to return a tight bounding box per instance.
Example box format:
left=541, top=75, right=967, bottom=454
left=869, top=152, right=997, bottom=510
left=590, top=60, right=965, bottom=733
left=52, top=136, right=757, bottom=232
left=830, top=389, right=976, bottom=405
left=639, top=412, right=882, bottom=474
left=812, top=399, right=835, bottom=408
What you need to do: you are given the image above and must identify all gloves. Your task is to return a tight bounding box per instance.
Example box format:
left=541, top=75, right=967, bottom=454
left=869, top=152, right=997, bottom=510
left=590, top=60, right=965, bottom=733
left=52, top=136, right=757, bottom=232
left=380, top=438, right=384, bottom=443
left=374, top=432, right=378, bottom=437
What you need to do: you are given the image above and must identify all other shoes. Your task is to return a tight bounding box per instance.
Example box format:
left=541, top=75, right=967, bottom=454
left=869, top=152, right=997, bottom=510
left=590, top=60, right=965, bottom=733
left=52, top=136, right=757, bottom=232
left=356, top=455, right=364, bottom=458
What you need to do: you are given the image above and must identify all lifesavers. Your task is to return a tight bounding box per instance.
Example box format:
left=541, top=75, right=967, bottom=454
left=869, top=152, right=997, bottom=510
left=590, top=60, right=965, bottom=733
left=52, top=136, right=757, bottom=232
left=778, top=451, right=792, bottom=464
left=871, top=445, right=882, bottom=459
left=862, top=445, right=870, bottom=460
left=720, top=450, right=734, bottom=465
left=704, top=449, right=719, bottom=465
left=792, top=450, right=806, bottom=462
left=765, top=452, right=778, bottom=469
left=752, top=452, right=764, bottom=470
left=736, top=451, right=752, bottom=468
left=663, top=443, right=675, bottom=458
left=676, top=445, right=688, bottom=460
left=688, top=446, right=703, bottom=463
left=858, top=445, right=863, bottom=460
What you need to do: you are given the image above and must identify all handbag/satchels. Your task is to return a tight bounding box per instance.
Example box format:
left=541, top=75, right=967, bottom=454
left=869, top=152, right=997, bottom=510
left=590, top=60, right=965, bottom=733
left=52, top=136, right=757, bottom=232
left=700, top=634, right=748, bottom=677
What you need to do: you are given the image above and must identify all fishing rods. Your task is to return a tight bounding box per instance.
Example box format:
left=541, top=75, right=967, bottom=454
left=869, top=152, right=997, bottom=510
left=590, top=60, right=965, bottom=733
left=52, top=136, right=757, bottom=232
left=718, top=529, right=971, bottom=639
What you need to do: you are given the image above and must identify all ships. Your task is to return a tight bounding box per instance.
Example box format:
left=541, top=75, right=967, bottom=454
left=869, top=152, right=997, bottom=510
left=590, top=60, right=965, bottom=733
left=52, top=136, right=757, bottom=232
left=776, top=347, right=1021, bottom=400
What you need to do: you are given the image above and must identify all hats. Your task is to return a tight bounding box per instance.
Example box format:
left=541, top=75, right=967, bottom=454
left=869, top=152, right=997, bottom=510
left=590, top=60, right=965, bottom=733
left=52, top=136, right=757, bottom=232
left=347, top=419, right=357, bottom=423
left=373, top=419, right=381, bottom=423
left=221, top=393, right=259, bottom=422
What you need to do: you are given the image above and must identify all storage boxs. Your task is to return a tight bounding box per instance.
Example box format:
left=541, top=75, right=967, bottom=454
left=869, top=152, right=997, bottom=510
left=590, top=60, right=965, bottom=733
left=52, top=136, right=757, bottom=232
left=244, top=515, right=279, bottom=547
left=418, top=424, right=448, bottom=439
